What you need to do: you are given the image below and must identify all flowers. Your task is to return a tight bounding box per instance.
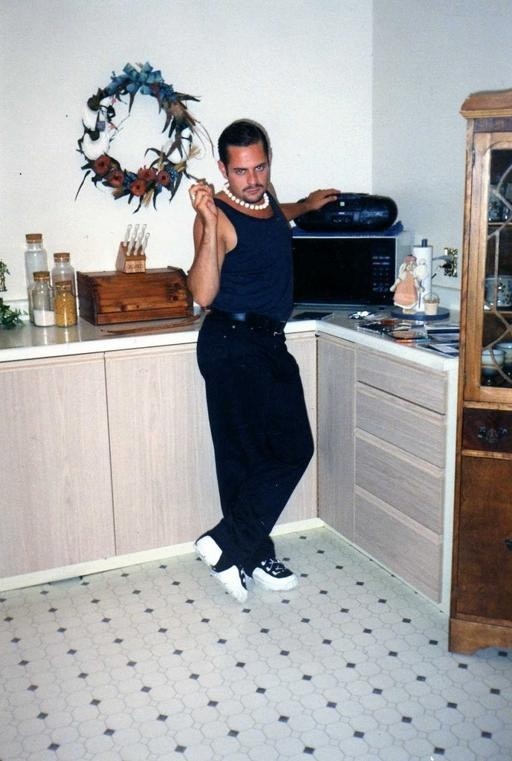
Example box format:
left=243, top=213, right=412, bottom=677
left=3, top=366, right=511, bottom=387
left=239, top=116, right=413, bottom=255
left=74, top=61, right=216, bottom=214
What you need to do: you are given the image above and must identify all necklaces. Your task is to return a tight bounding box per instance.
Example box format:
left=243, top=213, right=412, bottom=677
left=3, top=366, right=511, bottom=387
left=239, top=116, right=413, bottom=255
left=220, top=182, right=269, bottom=210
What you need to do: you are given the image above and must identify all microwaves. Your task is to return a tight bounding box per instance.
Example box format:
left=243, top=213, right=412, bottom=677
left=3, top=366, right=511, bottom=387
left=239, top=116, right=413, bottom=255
left=288, top=227, right=414, bottom=312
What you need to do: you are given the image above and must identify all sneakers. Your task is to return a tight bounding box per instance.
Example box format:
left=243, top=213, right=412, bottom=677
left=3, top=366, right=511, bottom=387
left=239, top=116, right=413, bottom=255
left=243, top=554, right=298, bottom=591
left=193, top=530, right=248, bottom=604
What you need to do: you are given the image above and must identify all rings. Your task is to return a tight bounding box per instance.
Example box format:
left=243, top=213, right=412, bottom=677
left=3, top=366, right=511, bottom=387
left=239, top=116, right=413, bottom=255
left=189, top=195, right=196, bottom=200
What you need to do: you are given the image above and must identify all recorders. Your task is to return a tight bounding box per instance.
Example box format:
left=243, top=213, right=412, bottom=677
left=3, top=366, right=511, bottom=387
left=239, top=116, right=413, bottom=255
left=293, top=193, right=397, bottom=231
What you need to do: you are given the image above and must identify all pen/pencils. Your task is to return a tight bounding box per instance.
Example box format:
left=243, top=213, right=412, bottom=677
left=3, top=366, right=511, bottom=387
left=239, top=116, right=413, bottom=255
left=393, top=338, right=432, bottom=342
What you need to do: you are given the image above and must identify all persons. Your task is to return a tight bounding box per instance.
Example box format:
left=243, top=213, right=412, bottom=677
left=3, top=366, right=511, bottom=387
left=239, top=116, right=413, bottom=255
left=185, top=119, right=343, bottom=603
left=391, top=256, right=421, bottom=311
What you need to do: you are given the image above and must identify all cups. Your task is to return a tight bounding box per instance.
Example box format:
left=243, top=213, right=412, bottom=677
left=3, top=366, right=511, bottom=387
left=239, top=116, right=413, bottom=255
left=489, top=184, right=503, bottom=221
left=484, top=274, right=512, bottom=307
left=496, top=343, right=512, bottom=377
left=482, top=350, right=505, bottom=386
left=503, top=183, right=512, bottom=222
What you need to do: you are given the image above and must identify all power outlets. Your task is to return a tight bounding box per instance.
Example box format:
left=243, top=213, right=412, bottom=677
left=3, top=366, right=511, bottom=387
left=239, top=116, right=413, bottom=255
left=443, top=247, right=459, bottom=279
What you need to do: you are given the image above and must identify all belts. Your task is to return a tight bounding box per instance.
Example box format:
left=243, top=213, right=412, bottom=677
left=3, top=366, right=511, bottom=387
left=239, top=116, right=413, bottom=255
left=229, top=310, right=288, bottom=336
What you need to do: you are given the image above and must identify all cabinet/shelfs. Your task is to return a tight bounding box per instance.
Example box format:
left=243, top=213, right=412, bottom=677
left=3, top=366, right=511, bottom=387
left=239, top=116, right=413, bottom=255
left=317, top=332, right=447, bottom=607
left=448, top=89, right=512, bottom=656
left=106, top=329, right=319, bottom=558
left=0, top=351, right=116, bottom=582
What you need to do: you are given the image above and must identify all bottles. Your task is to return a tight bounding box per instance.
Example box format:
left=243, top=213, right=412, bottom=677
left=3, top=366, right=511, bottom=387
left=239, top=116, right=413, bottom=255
left=23, top=234, right=48, bottom=324
left=53, top=280, right=78, bottom=327
left=52, top=253, right=74, bottom=281
left=28, top=272, right=55, bottom=327
